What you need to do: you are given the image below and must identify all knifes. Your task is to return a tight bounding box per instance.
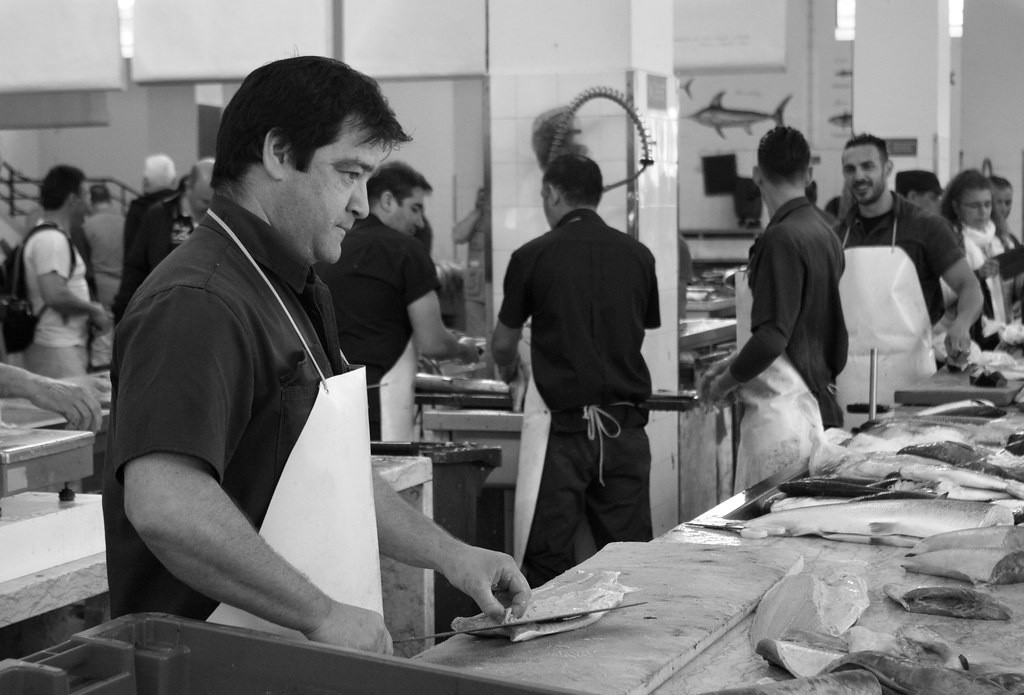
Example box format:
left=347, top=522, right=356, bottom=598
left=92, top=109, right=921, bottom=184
left=392, top=601, right=648, bottom=644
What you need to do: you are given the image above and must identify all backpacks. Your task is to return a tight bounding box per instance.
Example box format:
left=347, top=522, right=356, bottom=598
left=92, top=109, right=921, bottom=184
left=0, top=222, right=77, bottom=355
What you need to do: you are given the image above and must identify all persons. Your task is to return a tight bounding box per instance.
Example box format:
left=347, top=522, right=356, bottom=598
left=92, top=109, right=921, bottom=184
left=894, top=171, right=948, bottom=223
left=81, top=183, right=126, bottom=306
left=103, top=54, right=534, bottom=656
left=696, top=122, right=850, bottom=502
left=942, top=169, right=1016, bottom=353
left=123, top=154, right=180, bottom=267
left=117, top=158, right=218, bottom=314
left=0, top=363, right=105, bottom=438
left=315, top=157, right=483, bottom=446
left=489, top=154, right=662, bottom=593
left=980, top=176, right=1024, bottom=323
left=826, top=134, right=984, bottom=432
left=16, top=165, right=114, bottom=384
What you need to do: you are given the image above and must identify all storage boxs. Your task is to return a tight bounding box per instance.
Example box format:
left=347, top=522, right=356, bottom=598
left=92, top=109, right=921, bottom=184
left=0, top=610, right=603, bottom=695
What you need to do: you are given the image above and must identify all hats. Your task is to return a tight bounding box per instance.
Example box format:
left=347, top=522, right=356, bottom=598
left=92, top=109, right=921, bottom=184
left=894, top=171, right=944, bottom=198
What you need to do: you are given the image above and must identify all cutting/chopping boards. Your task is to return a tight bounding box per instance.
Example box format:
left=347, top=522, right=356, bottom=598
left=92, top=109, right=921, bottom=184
left=411, top=538, right=805, bottom=695
left=896, top=365, right=1022, bottom=405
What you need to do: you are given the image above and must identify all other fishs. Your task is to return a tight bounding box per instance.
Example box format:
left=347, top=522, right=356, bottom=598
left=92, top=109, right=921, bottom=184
left=704, top=398, right=1024, bottom=695
